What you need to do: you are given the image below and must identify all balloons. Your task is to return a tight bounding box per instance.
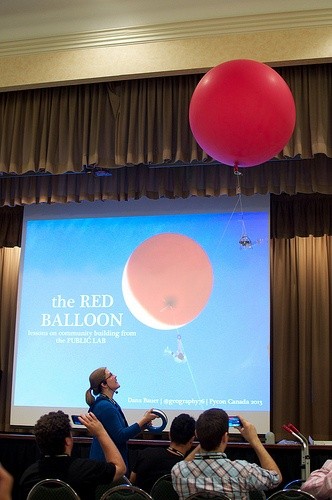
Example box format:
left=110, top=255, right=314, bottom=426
left=189, top=59, right=296, bottom=169
left=121, top=233, right=214, bottom=331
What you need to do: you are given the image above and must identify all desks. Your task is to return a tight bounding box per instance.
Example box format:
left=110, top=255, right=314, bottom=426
left=0, top=431, right=332, bottom=500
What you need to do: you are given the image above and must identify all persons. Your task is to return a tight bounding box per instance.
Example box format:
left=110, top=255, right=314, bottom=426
left=0, top=408, right=332, bottom=500
left=84, top=368, right=160, bottom=470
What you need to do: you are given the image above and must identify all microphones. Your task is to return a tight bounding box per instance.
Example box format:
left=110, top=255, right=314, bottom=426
left=107, top=383, right=118, bottom=394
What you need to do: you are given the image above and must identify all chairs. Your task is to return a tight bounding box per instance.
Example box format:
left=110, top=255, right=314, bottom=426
left=25, top=473, right=317, bottom=500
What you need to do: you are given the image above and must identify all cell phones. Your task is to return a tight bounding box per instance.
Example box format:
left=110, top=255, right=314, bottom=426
left=228, top=416, right=242, bottom=427
left=71, top=415, right=83, bottom=425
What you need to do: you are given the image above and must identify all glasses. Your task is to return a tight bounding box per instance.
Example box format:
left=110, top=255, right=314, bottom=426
left=106, top=372, right=113, bottom=379
